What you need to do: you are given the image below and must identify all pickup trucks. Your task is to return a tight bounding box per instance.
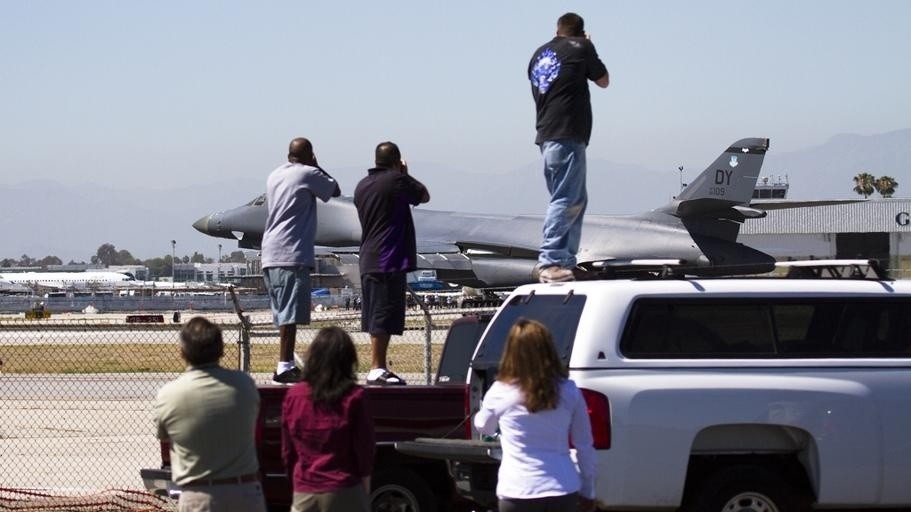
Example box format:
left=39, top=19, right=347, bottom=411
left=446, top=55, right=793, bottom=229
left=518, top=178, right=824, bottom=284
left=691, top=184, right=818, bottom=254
left=135, top=292, right=515, bottom=510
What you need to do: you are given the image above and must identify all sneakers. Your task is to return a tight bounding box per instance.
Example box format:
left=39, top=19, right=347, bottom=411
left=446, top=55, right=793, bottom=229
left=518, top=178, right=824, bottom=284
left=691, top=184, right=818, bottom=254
left=273, top=366, right=302, bottom=384
left=539, top=266, right=576, bottom=283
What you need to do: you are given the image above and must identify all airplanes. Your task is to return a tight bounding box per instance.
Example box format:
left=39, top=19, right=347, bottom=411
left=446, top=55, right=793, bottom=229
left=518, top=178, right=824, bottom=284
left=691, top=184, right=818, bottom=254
left=186, top=126, right=781, bottom=323
left=0, top=257, right=247, bottom=322
left=404, top=279, right=515, bottom=312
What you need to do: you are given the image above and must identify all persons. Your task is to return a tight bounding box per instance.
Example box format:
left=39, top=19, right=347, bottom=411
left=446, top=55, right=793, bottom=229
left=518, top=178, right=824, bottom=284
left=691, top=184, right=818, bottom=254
left=353, top=141, right=430, bottom=385
left=528, top=13, right=609, bottom=283
left=258, top=138, right=342, bottom=382
left=344, top=296, right=361, bottom=312
left=473, top=318, right=597, bottom=512
left=406, top=291, right=453, bottom=311
left=280, top=326, right=375, bottom=511
left=156, top=317, right=270, bottom=511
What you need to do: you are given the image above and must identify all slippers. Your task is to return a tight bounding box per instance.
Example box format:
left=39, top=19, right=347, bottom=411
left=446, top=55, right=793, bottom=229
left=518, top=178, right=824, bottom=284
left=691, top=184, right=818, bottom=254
left=367, top=369, right=407, bottom=385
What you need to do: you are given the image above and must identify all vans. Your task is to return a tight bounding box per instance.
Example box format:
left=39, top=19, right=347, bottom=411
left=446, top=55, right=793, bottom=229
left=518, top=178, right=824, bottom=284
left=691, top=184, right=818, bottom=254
left=457, top=252, right=911, bottom=511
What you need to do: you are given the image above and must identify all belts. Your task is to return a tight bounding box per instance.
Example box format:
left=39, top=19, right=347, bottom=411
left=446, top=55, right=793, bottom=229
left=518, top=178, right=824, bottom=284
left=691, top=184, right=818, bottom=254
left=187, top=473, right=255, bottom=486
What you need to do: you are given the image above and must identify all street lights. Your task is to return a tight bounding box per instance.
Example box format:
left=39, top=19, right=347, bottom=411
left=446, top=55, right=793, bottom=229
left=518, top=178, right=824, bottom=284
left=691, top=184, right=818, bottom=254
left=216, top=242, right=223, bottom=285
left=676, top=163, right=685, bottom=195
left=168, top=238, right=178, bottom=288
